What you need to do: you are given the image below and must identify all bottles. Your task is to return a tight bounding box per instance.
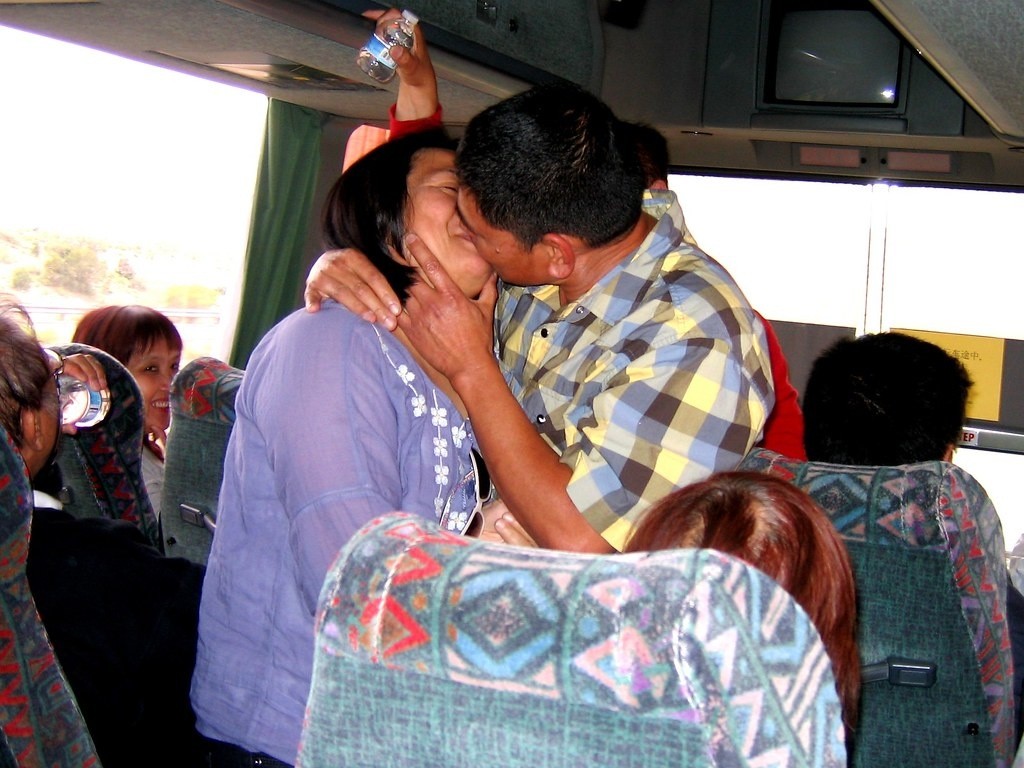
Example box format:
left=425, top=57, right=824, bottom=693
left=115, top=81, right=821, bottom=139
left=59, top=375, right=111, bottom=427
left=357, top=10, right=419, bottom=82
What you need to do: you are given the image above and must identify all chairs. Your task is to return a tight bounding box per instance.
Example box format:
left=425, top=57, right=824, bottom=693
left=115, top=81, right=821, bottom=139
left=0, top=426, right=105, bottom=768
left=734, top=448, right=1016, bottom=768
left=158, top=357, right=245, bottom=566
left=43, top=344, right=159, bottom=554
left=295, top=510, right=848, bottom=768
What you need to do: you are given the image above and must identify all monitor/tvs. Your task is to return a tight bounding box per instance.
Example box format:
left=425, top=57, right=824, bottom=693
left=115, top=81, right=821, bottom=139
left=756, top=0, right=911, bottom=116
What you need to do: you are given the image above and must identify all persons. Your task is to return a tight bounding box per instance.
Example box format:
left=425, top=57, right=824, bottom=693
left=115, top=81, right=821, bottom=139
left=300, top=84, right=775, bottom=559
left=0, top=5, right=1024, bottom=768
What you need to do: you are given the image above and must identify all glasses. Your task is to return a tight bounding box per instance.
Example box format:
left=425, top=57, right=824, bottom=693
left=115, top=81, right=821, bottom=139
left=38, top=348, right=64, bottom=392
